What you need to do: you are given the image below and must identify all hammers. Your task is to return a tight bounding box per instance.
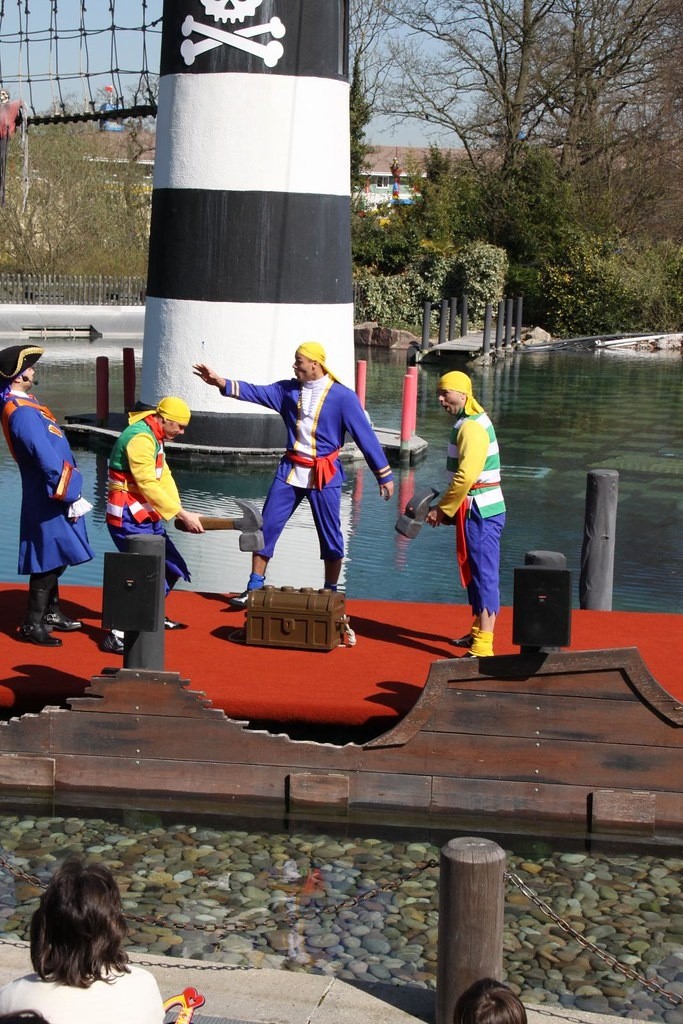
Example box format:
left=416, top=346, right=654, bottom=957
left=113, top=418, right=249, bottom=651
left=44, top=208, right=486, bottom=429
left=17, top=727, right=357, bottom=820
left=394, top=486, right=441, bottom=540
left=174, top=498, right=264, bottom=553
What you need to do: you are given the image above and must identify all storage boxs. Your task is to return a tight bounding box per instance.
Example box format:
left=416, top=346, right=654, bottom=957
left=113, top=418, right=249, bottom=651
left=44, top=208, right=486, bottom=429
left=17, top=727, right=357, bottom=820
left=244, top=585, right=352, bottom=651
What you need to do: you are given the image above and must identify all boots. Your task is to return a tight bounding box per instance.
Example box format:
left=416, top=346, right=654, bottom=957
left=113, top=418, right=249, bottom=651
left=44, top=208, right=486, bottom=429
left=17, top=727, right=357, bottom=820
left=20, top=587, right=85, bottom=647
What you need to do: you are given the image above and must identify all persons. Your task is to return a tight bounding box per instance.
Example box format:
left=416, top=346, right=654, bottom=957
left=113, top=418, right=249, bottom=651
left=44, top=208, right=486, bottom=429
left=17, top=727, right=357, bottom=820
left=0, top=861, right=168, bottom=1024
left=192, top=342, right=394, bottom=607
left=0, top=345, right=96, bottom=646
left=452, top=976, right=527, bottom=1024
left=424, top=371, right=506, bottom=659
left=98, top=396, right=206, bottom=655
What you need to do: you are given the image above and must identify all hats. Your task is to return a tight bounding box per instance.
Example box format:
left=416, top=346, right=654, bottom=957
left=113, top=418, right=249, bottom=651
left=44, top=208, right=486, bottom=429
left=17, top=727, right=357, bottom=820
left=1, top=344, right=43, bottom=384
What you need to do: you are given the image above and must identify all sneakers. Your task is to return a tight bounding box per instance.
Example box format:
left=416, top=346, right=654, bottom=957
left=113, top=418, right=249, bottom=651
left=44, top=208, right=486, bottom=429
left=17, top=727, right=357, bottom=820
left=449, top=633, right=473, bottom=646
left=164, top=616, right=181, bottom=630
left=229, top=591, right=251, bottom=607
left=102, top=633, right=128, bottom=653
